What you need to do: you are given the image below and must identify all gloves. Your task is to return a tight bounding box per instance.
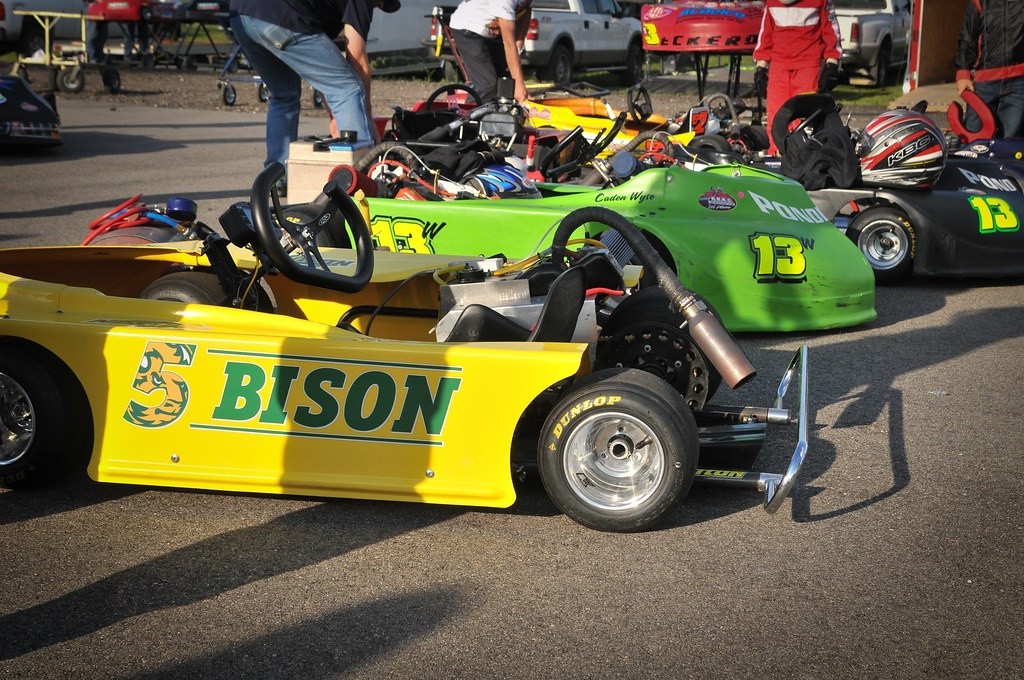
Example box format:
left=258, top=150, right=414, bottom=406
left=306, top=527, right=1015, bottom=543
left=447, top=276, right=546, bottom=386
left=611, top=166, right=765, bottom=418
left=818, top=62, right=838, bottom=93
left=754, top=66, right=768, bottom=100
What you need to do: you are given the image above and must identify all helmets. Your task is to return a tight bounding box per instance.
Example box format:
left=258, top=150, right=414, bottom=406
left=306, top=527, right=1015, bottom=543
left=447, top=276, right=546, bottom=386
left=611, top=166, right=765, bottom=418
left=855, top=110, right=948, bottom=186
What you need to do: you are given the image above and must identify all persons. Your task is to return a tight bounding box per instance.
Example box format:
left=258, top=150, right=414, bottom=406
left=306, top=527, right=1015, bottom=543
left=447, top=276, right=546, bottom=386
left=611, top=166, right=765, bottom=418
left=955, top=0, right=1024, bottom=141
left=229, top=0, right=402, bottom=197
left=83, top=0, right=108, bottom=63
left=449, top=0, right=533, bottom=106
left=753, top=0, right=843, bottom=156
left=123, top=24, right=148, bottom=61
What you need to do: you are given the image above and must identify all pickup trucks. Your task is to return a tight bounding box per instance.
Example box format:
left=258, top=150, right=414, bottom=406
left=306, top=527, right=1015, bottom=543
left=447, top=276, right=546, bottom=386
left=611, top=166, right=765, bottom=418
left=832, top=0, right=911, bottom=88
left=421, top=0, right=646, bottom=87
